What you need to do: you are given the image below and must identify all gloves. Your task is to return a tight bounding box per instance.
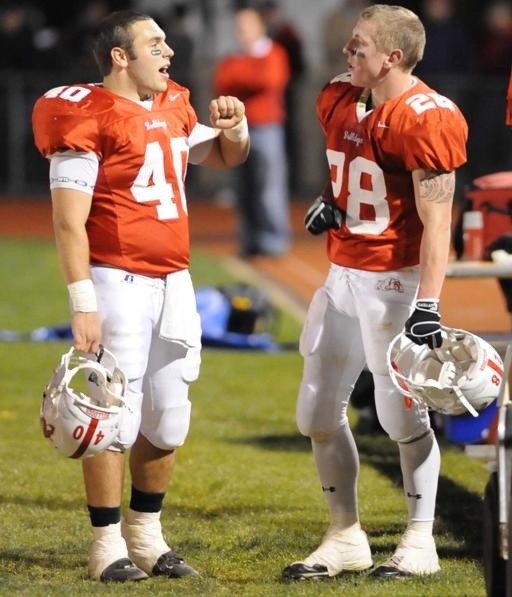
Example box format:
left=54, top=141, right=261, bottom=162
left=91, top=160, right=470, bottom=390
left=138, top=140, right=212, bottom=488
left=404, top=300, right=443, bottom=349
left=304, top=195, right=340, bottom=235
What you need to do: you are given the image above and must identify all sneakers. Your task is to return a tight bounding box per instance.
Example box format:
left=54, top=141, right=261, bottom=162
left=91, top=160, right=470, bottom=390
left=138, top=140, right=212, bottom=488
left=371, top=531, right=440, bottom=578
left=121, top=520, right=197, bottom=578
left=88, top=539, right=148, bottom=582
left=282, top=524, right=374, bottom=582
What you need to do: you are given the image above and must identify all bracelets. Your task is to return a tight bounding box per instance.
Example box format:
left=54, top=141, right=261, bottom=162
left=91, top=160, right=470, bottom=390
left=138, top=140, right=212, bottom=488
left=67, top=279, right=98, bottom=313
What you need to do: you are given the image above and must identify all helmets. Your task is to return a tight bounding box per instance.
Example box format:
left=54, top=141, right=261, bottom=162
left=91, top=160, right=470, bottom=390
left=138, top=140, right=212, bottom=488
left=387, top=326, right=504, bottom=417
left=41, top=346, right=127, bottom=459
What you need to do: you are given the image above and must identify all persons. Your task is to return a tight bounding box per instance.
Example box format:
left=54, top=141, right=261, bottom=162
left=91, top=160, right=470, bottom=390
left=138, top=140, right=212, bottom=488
left=1, top=0, right=511, bottom=260
left=280, top=4, right=469, bottom=582
left=32, top=9, right=251, bottom=585
